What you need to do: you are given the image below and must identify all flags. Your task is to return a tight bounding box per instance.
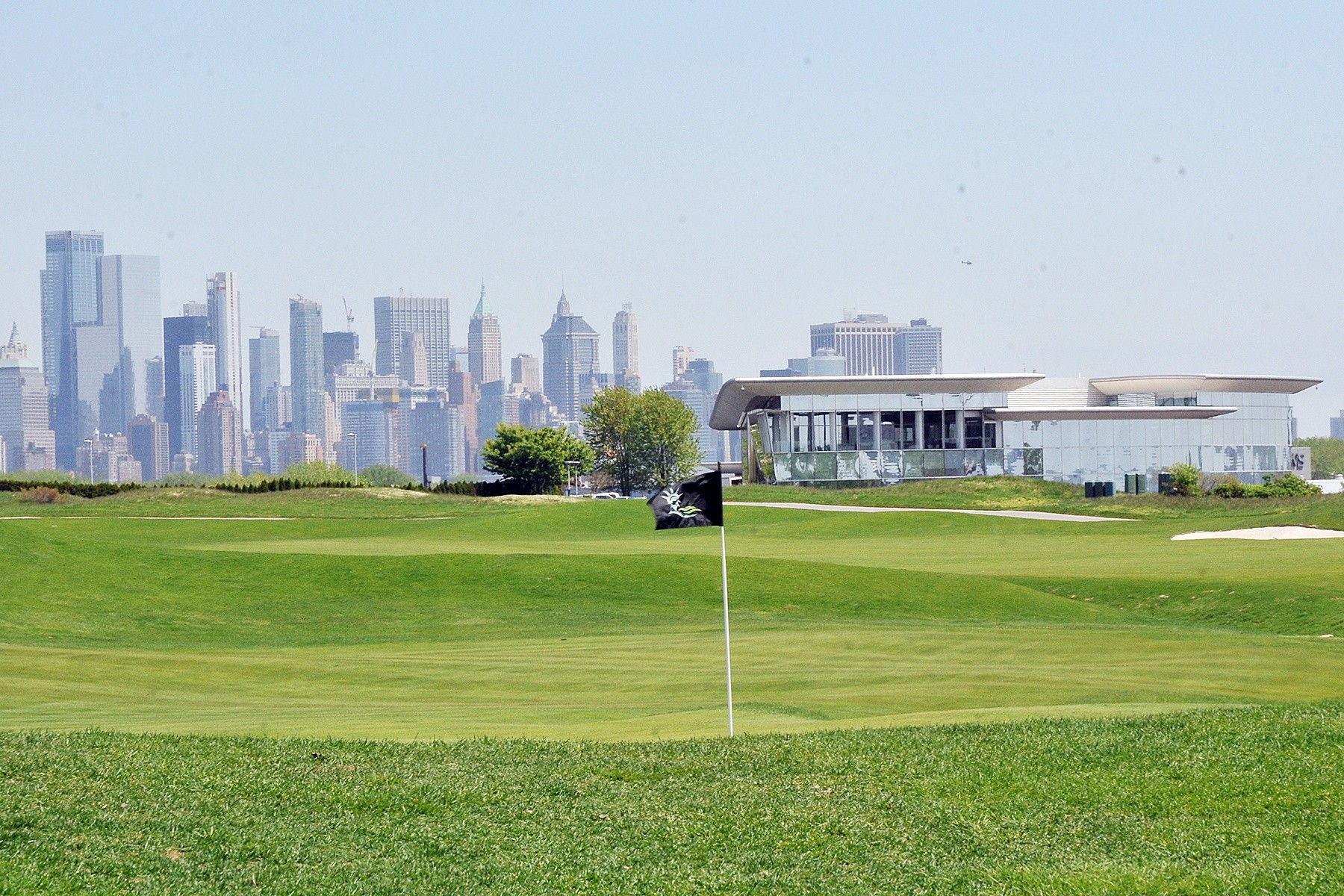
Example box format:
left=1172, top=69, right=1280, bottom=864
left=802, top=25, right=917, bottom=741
left=646, top=470, right=724, bottom=530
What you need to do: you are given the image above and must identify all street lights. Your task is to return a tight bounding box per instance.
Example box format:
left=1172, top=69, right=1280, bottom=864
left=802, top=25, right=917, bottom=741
left=84, top=439, right=94, bottom=485
left=347, top=433, right=357, bottom=486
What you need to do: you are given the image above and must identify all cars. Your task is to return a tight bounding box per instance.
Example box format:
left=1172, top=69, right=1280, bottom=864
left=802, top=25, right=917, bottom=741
left=569, top=492, right=647, bottom=500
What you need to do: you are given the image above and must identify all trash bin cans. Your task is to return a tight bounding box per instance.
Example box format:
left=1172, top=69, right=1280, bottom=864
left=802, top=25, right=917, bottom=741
left=1095, top=482, right=1105, bottom=497
left=1105, top=481, right=1116, bottom=497
left=1085, top=481, right=1097, bottom=498
